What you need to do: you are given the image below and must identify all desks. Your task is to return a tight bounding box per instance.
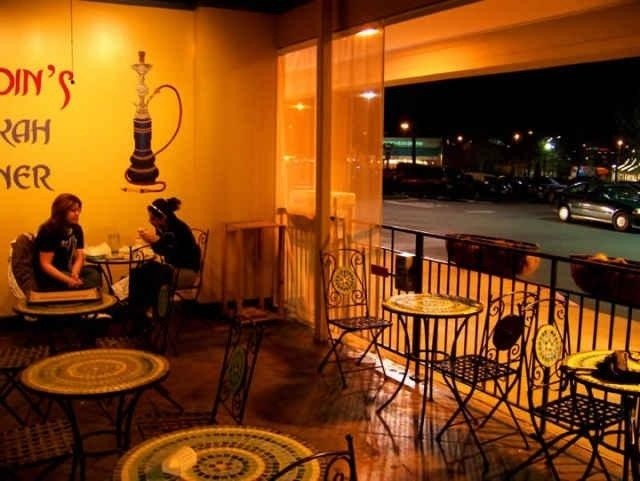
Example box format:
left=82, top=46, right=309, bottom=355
left=11, top=288, right=120, bottom=356
left=114, top=423, right=323, bottom=481
left=376, top=292, right=483, bottom=441
left=18, top=347, right=173, bottom=481
left=85, top=246, right=156, bottom=301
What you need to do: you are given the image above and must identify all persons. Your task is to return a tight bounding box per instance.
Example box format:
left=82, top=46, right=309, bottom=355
left=136, top=196, right=202, bottom=322
left=33, top=192, right=114, bottom=322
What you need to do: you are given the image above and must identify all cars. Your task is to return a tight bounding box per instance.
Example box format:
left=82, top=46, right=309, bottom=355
left=553, top=180, right=640, bottom=232
left=394, top=162, right=565, bottom=203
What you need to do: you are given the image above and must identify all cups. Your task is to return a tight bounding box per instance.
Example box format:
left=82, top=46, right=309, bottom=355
left=108, top=232, right=121, bottom=253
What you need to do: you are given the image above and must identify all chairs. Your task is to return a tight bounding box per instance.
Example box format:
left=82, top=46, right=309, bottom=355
left=515, top=293, right=639, bottom=481
left=127, top=229, right=213, bottom=354
left=7, top=227, right=99, bottom=358
left=132, top=309, right=266, bottom=443
left=315, top=244, right=392, bottom=387
left=2, top=344, right=52, bottom=428
left=433, top=291, right=541, bottom=462
left=271, top=432, right=356, bottom=481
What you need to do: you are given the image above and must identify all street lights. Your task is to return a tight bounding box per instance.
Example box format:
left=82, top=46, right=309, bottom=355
left=400, top=122, right=416, bottom=165
left=615, top=140, right=624, bottom=184
